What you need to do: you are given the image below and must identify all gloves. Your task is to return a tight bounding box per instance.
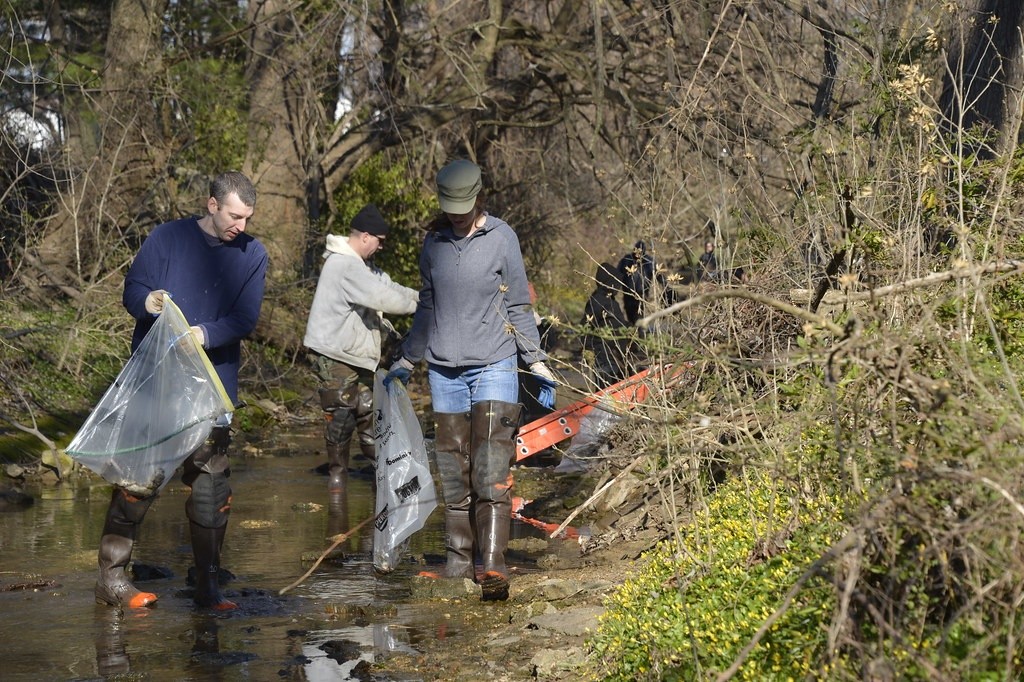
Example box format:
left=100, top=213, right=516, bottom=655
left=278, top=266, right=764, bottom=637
left=179, top=324, right=204, bottom=354
left=530, top=361, right=557, bottom=408
left=384, top=356, right=415, bottom=396
left=145, top=290, right=174, bottom=314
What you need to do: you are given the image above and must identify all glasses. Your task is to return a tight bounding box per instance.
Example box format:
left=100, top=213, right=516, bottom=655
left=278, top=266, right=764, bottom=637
left=372, top=234, right=386, bottom=243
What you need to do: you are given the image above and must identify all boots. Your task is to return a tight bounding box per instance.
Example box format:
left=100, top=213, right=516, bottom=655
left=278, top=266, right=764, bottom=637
left=470, top=400, right=522, bottom=595
left=433, top=409, right=477, bottom=587
left=319, top=389, right=351, bottom=493
left=187, top=519, right=238, bottom=611
left=94, top=486, right=158, bottom=608
left=348, top=390, right=375, bottom=472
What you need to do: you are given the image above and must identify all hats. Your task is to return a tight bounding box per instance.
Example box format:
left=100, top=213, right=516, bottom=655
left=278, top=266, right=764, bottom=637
left=353, top=203, right=389, bottom=237
left=436, top=160, right=482, bottom=214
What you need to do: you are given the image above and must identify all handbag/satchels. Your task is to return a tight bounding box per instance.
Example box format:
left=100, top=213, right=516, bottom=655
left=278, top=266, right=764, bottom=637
left=66, top=294, right=236, bottom=500
left=375, top=369, right=439, bottom=573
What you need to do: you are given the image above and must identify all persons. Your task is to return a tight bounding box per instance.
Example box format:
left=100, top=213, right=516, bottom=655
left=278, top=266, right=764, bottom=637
left=383, top=158, right=558, bottom=600
left=696, top=241, right=721, bottom=286
left=580, top=263, right=635, bottom=390
left=301, top=203, right=420, bottom=497
left=515, top=281, right=555, bottom=467
left=616, top=241, right=668, bottom=337
left=94, top=172, right=268, bottom=611
